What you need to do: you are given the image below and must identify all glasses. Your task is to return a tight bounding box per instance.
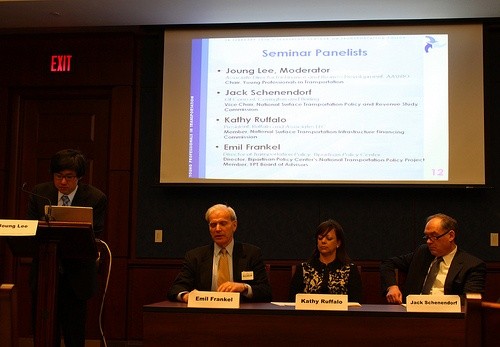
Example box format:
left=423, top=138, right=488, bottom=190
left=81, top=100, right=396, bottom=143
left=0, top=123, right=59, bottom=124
left=422, top=229, right=450, bottom=244
left=54, top=172, right=79, bottom=181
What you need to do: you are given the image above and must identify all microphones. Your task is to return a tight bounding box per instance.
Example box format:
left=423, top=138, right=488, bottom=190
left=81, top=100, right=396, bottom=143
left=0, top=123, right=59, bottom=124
left=21, top=182, right=51, bottom=218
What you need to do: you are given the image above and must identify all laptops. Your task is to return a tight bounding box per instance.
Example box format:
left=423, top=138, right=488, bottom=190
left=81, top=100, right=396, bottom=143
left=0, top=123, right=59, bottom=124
left=44, top=205, right=93, bottom=229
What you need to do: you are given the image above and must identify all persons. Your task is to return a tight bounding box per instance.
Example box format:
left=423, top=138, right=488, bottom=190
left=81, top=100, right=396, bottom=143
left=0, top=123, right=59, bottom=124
left=167, top=204, right=273, bottom=303
left=26, top=147, right=110, bottom=347
left=287, top=219, right=364, bottom=304
left=379, top=213, right=488, bottom=305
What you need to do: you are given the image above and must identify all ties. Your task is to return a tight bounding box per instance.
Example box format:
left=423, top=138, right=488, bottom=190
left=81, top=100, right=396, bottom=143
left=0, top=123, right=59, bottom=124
left=218, top=249, right=230, bottom=288
left=421, top=256, right=444, bottom=296
left=60, top=195, right=70, bottom=206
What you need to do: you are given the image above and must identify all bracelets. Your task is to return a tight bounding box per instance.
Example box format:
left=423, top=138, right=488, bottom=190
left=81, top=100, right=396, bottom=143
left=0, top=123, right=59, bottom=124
left=241, top=282, right=248, bottom=295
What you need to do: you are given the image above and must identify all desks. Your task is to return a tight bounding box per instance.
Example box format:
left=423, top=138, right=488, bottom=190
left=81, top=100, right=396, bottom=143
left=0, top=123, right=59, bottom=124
left=142, top=301, right=500, bottom=347
